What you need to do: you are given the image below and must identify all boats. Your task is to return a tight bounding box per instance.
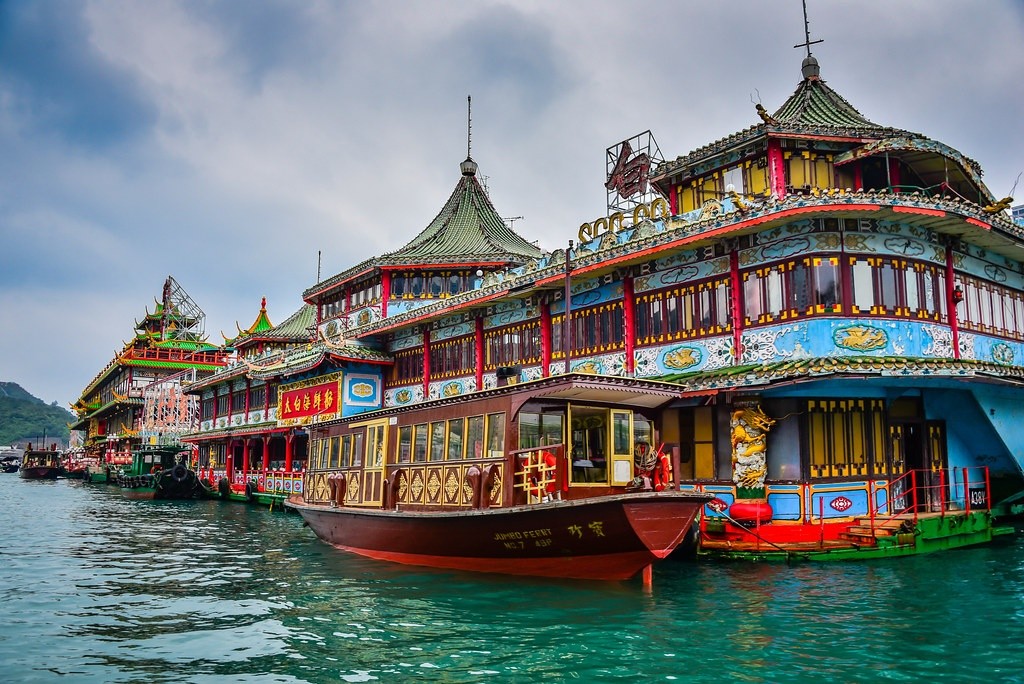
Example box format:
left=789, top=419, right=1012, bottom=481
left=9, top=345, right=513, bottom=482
left=0, top=427, right=108, bottom=484
left=116, top=443, right=190, bottom=501
left=282, top=366, right=717, bottom=584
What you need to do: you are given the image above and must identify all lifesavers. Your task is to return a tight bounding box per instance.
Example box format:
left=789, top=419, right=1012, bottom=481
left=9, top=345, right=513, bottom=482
left=155, top=472, right=161, bottom=483
left=245, top=482, right=256, bottom=501
left=653, top=452, right=670, bottom=492
left=119, top=475, right=148, bottom=489
left=87, top=474, right=92, bottom=482
left=521, top=450, right=559, bottom=497
left=218, top=480, right=227, bottom=497
left=83, top=471, right=87, bottom=480
left=171, top=465, right=189, bottom=482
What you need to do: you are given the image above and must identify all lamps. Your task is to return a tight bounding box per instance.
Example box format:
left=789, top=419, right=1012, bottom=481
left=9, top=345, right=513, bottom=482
left=497, top=366, right=517, bottom=380
left=953, top=285, right=964, bottom=303
